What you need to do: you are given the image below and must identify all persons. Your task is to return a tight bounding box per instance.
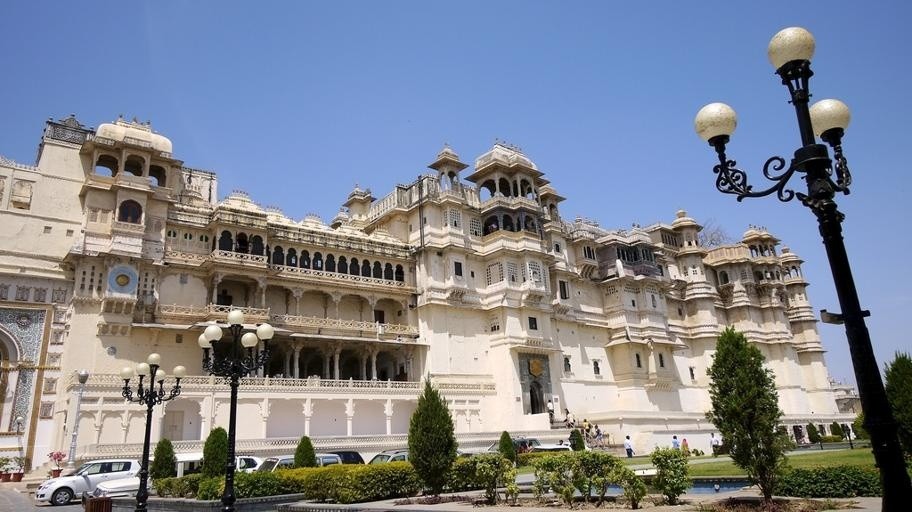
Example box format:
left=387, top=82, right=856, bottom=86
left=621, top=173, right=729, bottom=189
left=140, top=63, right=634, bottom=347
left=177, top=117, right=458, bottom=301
left=624, top=435, right=635, bottom=458
left=546, top=400, right=554, bottom=417
left=672, top=435, right=680, bottom=448
left=514, top=442, right=535, bottom=453
left=710, top=432, right=719, bottom=452
left=559, top=439, right=564, bottom=445
left=681, top=439, right=688, bottom=449
left=564, top=408, right=575, bottom=428
left=582, top=419, right=603, bottom=442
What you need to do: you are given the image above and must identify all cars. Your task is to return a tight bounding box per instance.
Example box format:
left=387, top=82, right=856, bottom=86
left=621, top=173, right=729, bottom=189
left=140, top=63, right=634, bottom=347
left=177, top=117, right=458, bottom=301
left=234, top=452, right=262, bottom=475
left=32, top=457, right=147, bottom=508
left=366, top=450, right=412, bottom=468
left=452, top=435, right=592, bottom=459
left=325, top=450, right=364, bottom=467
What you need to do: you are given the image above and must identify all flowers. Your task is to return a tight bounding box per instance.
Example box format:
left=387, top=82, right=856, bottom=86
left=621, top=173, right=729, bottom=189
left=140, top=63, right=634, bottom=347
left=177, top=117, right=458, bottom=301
left=48, top=451, right=66, bottom=469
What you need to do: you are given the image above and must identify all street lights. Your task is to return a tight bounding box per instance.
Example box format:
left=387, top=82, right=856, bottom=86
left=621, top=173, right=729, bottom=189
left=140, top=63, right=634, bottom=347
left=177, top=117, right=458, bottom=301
left=114, top=349, right=191, bottom=512
left=693, top=25, right=912, bottom=512
left=59, top=367, right=94, bottom=476
left=195, top=305, right=276, bottom=512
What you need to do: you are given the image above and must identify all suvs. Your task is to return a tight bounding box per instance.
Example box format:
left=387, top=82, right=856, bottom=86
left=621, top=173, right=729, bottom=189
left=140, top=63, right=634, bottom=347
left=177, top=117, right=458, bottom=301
left=78, top=450, right=204, bottom=509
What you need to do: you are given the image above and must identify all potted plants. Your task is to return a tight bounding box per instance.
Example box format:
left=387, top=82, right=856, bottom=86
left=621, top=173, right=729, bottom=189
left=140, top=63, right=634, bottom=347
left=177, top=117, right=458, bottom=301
left=13, top=456, right=32, bottom=482
left=0, top=456, right=12, bottom=481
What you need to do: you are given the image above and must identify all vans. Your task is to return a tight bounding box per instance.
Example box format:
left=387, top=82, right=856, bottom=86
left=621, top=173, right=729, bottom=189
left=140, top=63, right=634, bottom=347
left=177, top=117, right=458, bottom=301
left=253, top=453, right=343, bottom=474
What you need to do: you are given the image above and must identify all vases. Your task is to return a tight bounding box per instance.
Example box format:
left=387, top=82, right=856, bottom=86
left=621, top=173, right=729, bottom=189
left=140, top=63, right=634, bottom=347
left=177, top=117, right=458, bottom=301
left=51, top=470, right=61, bottom=477
